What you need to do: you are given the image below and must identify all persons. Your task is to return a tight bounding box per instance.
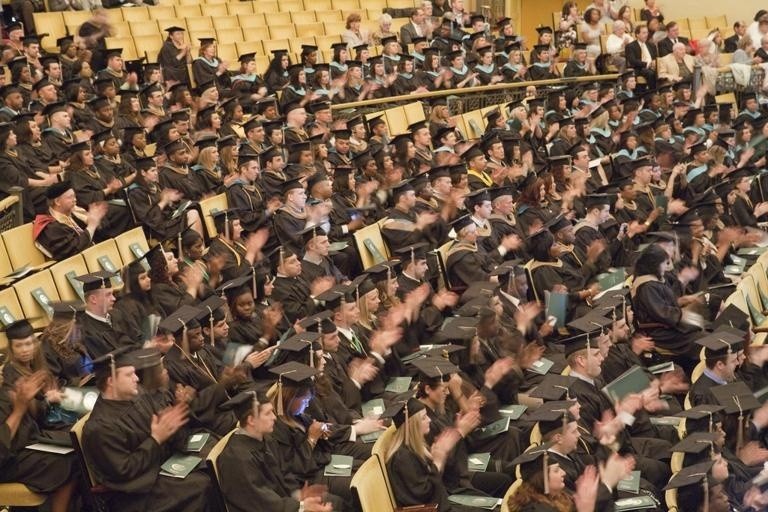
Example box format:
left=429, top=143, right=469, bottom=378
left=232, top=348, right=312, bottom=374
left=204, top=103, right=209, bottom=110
left=1, top=1, right=768, bottom=512
